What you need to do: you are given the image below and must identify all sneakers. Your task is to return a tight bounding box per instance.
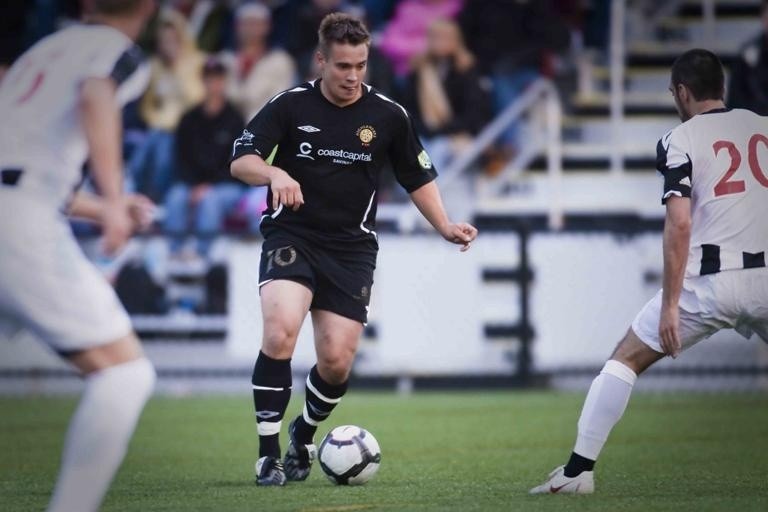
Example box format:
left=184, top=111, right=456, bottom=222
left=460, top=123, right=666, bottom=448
left=255, top=457, right=286, bottom=486
left=527, top=465, right=594, bottom=494
left=285, top=415, right=318, bottom=482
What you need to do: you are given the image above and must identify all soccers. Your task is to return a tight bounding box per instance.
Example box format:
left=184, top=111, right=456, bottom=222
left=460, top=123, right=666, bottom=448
left=318, top=425, right=381, bottom=484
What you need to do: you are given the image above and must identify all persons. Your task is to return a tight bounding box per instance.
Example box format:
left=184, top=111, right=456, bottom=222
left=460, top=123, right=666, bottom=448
left=111, top=3, right=294, bottom=235
left=309, top=3, right=479, bottom=137
left=230, top=12, right=479, bottom=485
left=530, top=49, right=768, bottom=495
left=0, top=0, right=157, bottom=512
